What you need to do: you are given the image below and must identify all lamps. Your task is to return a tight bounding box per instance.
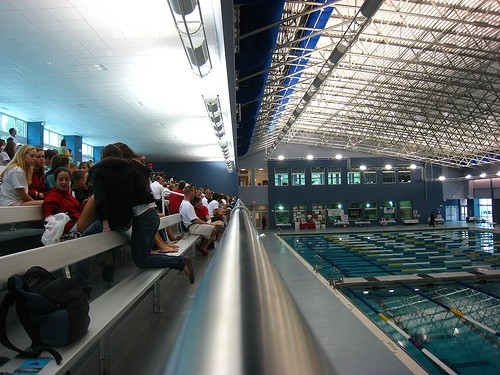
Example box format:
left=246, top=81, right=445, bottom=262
left=265, top=0, right=385, bottom=159
left=167, top=0, right=234, bottom=173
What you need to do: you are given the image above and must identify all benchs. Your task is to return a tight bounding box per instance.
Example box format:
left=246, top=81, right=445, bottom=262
left=332, top=222, right=349, bottom=228
left=380, top=221, right=396, bottom=226
left=0, top=201, right=200, bottom=375
left=276, top=223, right=291, bottom=226
left=354, top=221, right=370, bottom=227
left=427, top=218, right=444, bottom=223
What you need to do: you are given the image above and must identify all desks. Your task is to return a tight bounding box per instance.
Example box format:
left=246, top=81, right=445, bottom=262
left=404, top=219, right=418, bottom=223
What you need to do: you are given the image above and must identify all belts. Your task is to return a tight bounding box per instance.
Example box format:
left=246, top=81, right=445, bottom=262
left=187, top=223, right=193, bottom=230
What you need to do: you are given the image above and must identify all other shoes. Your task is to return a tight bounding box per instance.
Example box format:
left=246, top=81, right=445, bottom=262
left=198, top=244, right=209, bottom=256
left=184, top=256, right=195, bottom=285
left=177, top=233, right=185, bottom=240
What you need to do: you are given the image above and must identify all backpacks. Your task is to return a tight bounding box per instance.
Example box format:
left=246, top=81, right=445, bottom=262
left=0, top=266, right=90, bottom=365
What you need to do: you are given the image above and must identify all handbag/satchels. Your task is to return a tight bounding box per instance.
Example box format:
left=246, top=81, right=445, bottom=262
left=41, top=212, right=69, bottom=246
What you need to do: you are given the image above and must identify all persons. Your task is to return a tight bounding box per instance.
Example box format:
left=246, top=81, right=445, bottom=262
left=0, top=128, right=236, bottom=256
left=262, top=216, right=266, bottom=229
left=74, top=144, right=134, bottom=286
left=112, top=142, right=194, bottom=284
left=429, top=212, right=435, bottom=227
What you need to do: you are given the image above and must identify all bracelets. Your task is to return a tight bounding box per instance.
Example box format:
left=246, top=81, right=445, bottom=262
left=103, top=228, right=110, bottom=232
